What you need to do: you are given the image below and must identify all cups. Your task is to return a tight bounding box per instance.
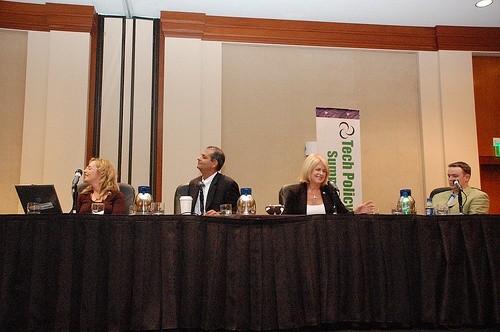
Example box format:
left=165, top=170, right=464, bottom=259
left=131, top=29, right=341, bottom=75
left=27, top=202, right=41, bottom=214
left=129, top=201, right=136, bottom=214
left=179, top=196, right=193, bottom=215
left=220, top=204, right=232, bottom=215
left=92, top=203, right=104, bottom=214
left=436, top=203, right=449, bottom=215
left=150, top=202, right=165, bottom=215
left=391, top=201, right=401, bottom=214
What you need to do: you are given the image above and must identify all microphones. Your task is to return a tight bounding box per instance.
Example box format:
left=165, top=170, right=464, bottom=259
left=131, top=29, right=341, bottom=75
left=72, top=169, right=82, bottom=187
left=453, top=180, right=463, bottom=192
left=198, top=181, right=203, bottom=187
left=328, top=180, right=339, bottom=191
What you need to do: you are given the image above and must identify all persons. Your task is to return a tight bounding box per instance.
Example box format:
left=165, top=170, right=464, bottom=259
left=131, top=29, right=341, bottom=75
left=70, top=158, right=127, bottom=215
left=432, top=162, right=489, bottom=214
left=188, top=146, right=240, bottom=215
left=281, top=153, right=376, bottom=214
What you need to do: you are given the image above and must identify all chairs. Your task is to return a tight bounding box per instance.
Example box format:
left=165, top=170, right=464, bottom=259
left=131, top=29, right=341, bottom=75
left=174, top=184, right=189, bottom=215
left=278, top=184, right=337, bottom=215
left=73, top=182, right=135, bottom=215
left=429, top=186, right=481, bottom=202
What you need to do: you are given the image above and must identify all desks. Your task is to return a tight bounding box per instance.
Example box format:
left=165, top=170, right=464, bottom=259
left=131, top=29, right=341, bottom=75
left=0, top=214, right=500, bottom=332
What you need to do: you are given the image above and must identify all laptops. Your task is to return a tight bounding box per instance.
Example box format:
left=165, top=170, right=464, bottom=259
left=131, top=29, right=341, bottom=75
left=15, top=184, right=63, bottom=214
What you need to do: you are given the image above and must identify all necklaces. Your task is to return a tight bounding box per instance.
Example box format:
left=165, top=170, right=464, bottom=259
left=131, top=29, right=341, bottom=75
left=308, top=187, right=320, bottom=200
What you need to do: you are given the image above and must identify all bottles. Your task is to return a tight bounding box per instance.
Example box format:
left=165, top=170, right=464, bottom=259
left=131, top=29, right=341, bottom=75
left=424, top=198, right=435, bottom=215
left=401, top=192, right=411, bottom=214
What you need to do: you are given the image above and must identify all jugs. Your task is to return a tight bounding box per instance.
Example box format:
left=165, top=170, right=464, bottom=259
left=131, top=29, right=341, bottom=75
left=236, top=187, right=256, bottom=215
left=135, top=186, right=153, bottom=215
left=397, top=189, right=416, bottom=214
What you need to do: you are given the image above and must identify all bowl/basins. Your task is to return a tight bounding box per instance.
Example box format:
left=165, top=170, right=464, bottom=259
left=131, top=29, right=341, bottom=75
left=265, top=204, right=284, bottom=215
